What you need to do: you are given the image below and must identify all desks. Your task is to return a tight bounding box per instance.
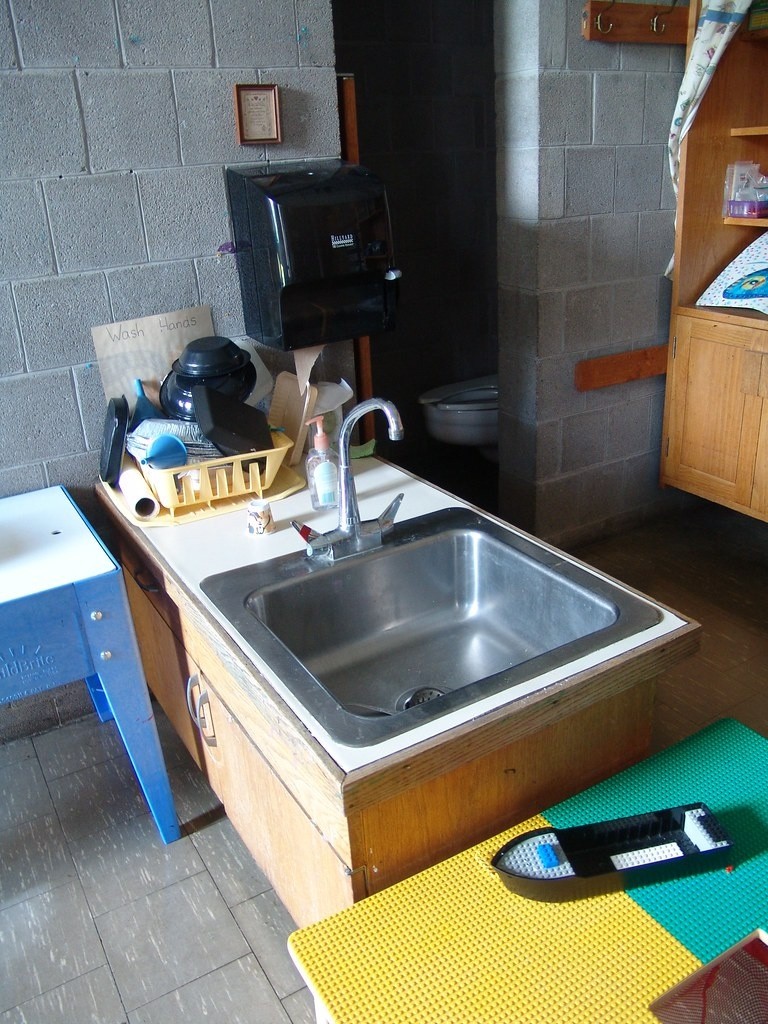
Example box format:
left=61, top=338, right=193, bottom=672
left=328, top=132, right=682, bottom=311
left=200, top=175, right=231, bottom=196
left=0, top=484, right=182, bottom=846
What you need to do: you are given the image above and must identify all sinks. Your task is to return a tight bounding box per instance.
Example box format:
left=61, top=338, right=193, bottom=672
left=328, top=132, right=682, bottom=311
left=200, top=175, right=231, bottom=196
left=200, top=501, right=663, bottom=750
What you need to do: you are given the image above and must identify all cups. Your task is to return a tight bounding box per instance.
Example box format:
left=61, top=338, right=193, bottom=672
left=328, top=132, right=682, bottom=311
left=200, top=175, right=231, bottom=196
left=247, top=501, right=278, bottom=537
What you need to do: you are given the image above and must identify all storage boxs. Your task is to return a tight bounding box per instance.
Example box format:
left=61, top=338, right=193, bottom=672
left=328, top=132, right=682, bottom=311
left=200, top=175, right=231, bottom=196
left=727, top=200, right=768, bottom=218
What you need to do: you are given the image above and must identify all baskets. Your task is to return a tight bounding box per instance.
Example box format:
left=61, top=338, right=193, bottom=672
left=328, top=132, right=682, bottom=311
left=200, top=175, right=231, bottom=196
left=135, top=418, right=294, bottom=516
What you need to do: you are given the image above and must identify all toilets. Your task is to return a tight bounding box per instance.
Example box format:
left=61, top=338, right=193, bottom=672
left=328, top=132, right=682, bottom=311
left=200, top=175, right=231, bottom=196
left=418, top=364, right=504, bottom=452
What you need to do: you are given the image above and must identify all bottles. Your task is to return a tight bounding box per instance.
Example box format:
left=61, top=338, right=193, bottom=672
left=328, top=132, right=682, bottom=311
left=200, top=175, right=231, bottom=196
left=305, top=416, right=339, bottom=510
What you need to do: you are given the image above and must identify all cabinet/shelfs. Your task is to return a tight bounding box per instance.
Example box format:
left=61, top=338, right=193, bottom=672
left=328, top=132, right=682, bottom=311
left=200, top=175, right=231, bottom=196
left=96, top=534, right=703, bottom=929
left=659, top=0, right=768, bottom=526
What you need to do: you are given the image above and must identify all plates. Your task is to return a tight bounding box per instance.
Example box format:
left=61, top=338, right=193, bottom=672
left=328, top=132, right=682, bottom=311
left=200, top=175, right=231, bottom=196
left=212, top=442, right=264, bottom=475
left=101, top=395, right=130, bottom=488
left=172, top=336, right=251, bottom=378
left=159, top=361, right=256, bottom=421
left=191, top=385, right=275, bottom=463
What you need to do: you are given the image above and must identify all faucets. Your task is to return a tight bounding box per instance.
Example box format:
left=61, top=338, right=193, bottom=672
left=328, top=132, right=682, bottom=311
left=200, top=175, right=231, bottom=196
left=336, top=396, right=406, bottom=534
